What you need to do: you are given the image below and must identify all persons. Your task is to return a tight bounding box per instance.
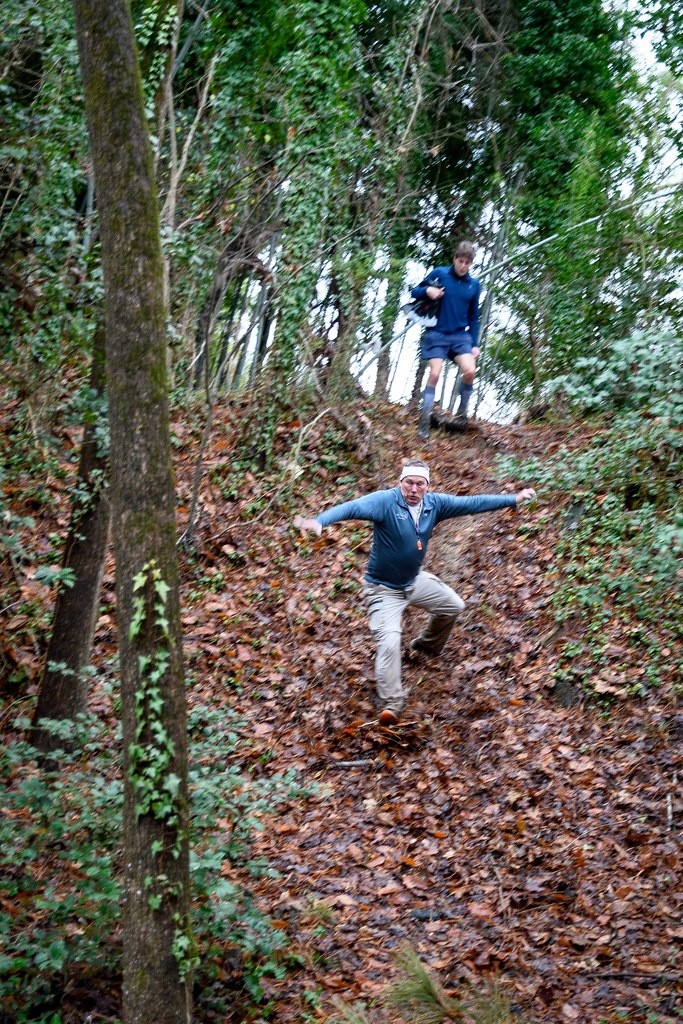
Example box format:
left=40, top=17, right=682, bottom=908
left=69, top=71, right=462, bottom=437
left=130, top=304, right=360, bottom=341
left=294, top=459, right=537, bottom=726
left=411, top=241, right=482, bottom=438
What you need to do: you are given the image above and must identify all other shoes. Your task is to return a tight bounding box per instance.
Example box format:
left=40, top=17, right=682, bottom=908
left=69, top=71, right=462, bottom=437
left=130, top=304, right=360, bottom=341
left=418, top=418, right=431, bottom=440
left=457, top=410, right=468, bottom=421
left=379, top=708, right=400, bottom=723
left=411, top=638, right=440, bottom=657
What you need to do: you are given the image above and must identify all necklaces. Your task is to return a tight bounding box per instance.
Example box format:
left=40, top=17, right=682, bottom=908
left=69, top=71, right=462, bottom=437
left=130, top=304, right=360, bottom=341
left=417, top=503, right=422, bottom=550
